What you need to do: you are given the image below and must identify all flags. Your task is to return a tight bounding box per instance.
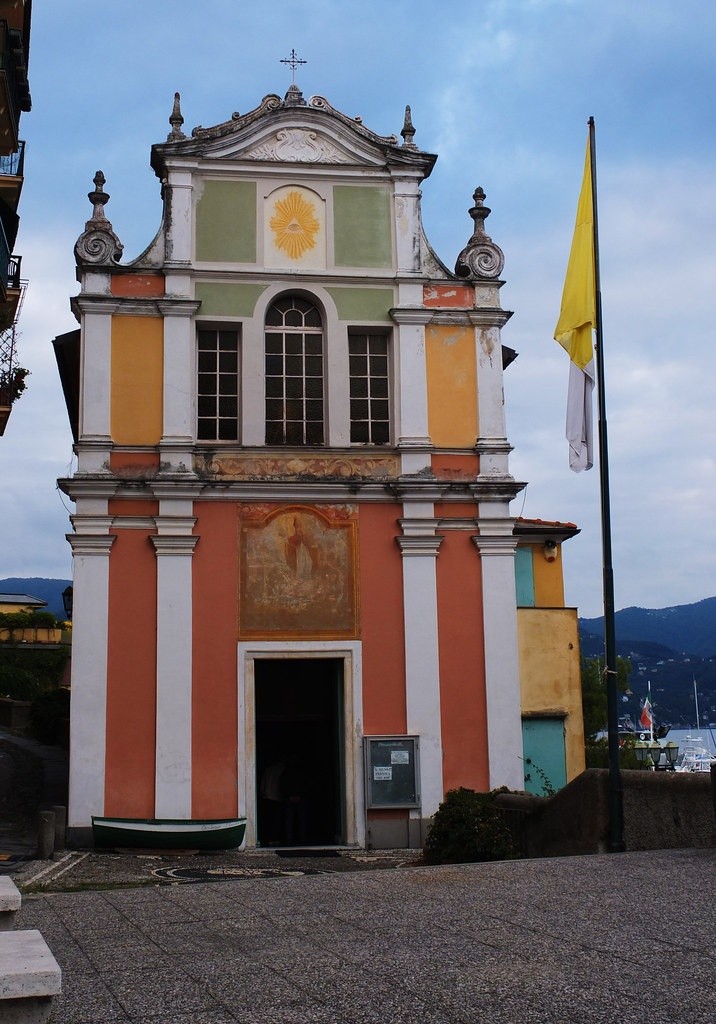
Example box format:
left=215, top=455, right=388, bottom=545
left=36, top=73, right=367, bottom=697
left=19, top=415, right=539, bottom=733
left=553, top=132, right=598, bottom=474
left=641, top=690, right=653, bottom=728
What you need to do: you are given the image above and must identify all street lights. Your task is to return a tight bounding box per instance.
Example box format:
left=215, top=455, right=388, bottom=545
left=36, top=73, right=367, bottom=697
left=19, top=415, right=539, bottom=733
left=633, top=742, right=679, bottom=771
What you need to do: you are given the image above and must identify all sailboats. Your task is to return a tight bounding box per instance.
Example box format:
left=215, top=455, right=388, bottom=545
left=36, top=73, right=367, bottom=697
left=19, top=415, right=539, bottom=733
left=672, top=673, right=716, bottom=775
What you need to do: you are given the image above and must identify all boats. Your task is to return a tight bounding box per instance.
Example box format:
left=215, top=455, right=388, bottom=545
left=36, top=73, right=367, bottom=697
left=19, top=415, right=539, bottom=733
left=589, top=680, right=671, bottom=746
